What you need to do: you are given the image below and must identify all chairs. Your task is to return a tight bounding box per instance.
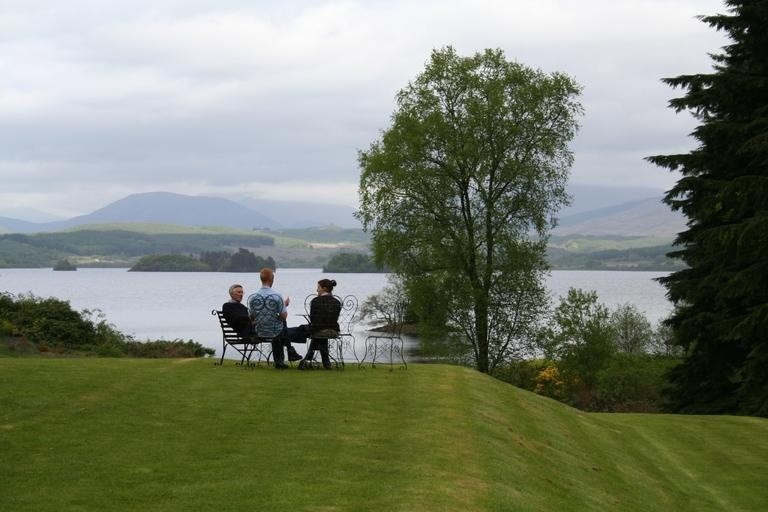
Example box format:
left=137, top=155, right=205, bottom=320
left=244, top=294, right=288, bottom=373
left=293, top=293, right=411, bottom=371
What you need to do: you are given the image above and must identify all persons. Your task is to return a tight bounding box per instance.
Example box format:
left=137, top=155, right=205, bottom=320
left=223, top=283, right=310, bottom=344
left=297, top=279, right=343, bottom=369
left=247, top=267, right=303, bottom=369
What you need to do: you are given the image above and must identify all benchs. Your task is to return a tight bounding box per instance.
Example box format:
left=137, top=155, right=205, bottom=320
left=210, top=307, right=268, bottom=369
